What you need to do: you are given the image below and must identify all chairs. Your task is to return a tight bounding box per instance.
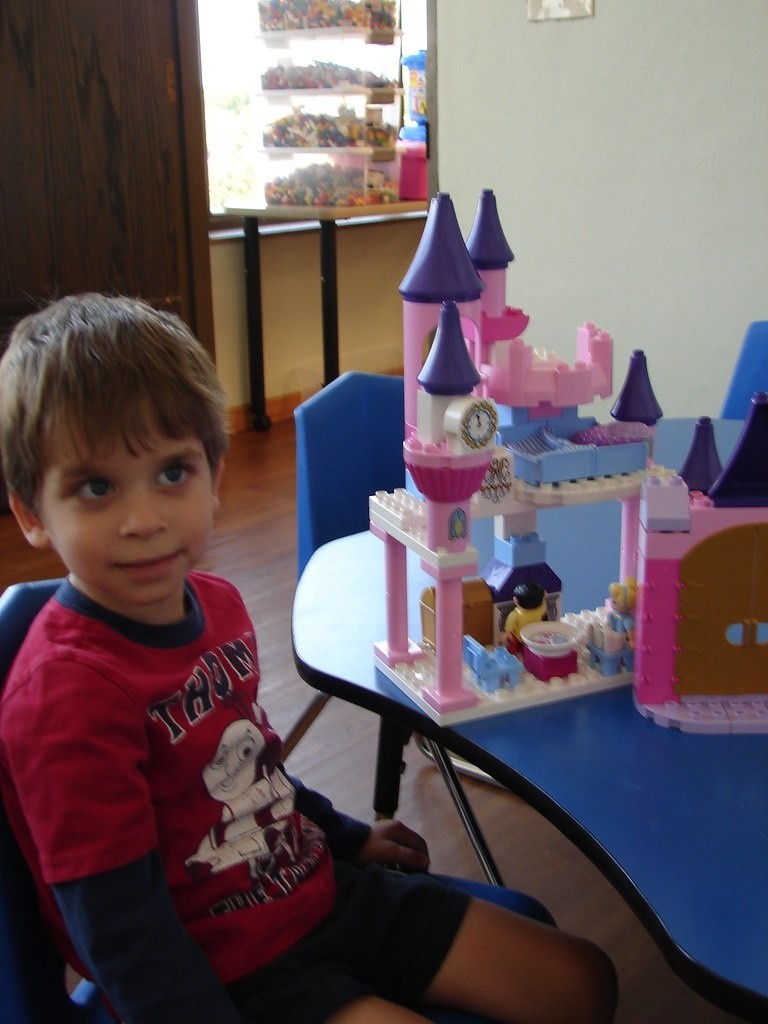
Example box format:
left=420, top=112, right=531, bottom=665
left=286, top=369, right=403, bottom=766
left=0, top=578, right=558, bottom=1024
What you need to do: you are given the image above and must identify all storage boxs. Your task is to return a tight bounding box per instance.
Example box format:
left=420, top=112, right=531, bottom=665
left=253, top=29, right=403, bottom=89
left=255, top=148, right=401, bottom=205
left=404, top=52, right=425, bottom=125
left=253, top=89, right=401, bottom=147
left=256, top=0, right=401, bottom=31
left=332, top=140, right=428, bottom=202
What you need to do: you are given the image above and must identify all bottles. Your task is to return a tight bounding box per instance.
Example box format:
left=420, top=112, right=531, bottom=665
left=401, top=50, right=428, bottom=124
left=397, top=127, right=428, bottom=199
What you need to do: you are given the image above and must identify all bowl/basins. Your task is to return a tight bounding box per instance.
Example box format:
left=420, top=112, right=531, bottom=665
left=521, top=620, right=580, bottom=657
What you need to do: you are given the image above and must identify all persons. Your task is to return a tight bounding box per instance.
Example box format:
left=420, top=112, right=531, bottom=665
left=0, top=292, right=621, bottom=1024
left=501, top=583, right=547, bottom=653
left=608, top=578, right=635, bottom=650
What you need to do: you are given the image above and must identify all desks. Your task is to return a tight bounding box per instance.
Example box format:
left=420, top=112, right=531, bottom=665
left=224, top=199, right=436, bottom=432
left=289, top=497, right=768, bottom=1024
